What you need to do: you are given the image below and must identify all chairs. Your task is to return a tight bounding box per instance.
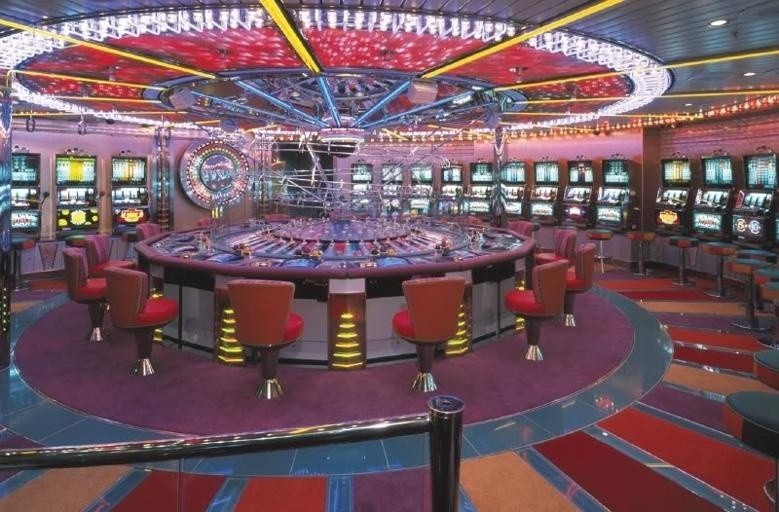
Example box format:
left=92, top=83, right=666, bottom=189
left=84, top=235, right=137, bottom=277
left=196, top=215, right=216, bottom=227
left=505, top=259, right=571, bottom=362
left=507, top=220, right=534, bottom=237
left=61, top=247, right=107, bottom=343
left=393, top=276, right=467, bottom=394
left=564, top=242, right=596, bottom=327
left=135, top=223, right=162, bottom=240
left=224, top=278, right=305, bottom=399
left=103, top=266, right=180, bottom=378
left=535, top=229, right=576, bottom=268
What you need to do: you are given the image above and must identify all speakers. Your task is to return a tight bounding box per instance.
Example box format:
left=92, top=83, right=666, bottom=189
left=408, top=80, right=437, bottom=105
left=169, top=87, right=195, bottom=111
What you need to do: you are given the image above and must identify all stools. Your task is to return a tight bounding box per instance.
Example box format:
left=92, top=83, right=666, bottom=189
left=588, top=228, right=613, bottom=278
left=725, top=249, right=777, bottom=511
left=668, top=237, right=700, bottom=286
left=625, top=230, right=656, bottom=277
left=705, top=242, right=738, bottom=299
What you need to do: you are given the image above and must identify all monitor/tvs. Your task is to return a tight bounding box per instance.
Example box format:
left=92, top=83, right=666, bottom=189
left=411, top=164, right=433, bottom=183
left=470, top=163, right=494, bottom=183
left=568, top=160, right=593, bottom=186
left=701, top=156, right=734, bottom=190
left=381, top=164, right=403, bottom=183
left=500, top=161, right=526, bottom=185
left=55, top=154, right=96, bottom=186
left=743, top=154, right=779, bottom=190
left=661, top=158, right=693, bottom=187
left=534, top=162, right=559, bottom=185
left=602, top=160, right=630, bottom=187
left=441, top=163, right=463, bottom=183
left=11, top=152, right=40, bottom=187
left=111, top=156, right=147, bottom=185
left=351, top=163, right=373, bottom=183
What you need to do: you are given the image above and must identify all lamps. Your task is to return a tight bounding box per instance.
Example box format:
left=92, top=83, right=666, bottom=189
left=1, top=0, right=674, bottom=146
